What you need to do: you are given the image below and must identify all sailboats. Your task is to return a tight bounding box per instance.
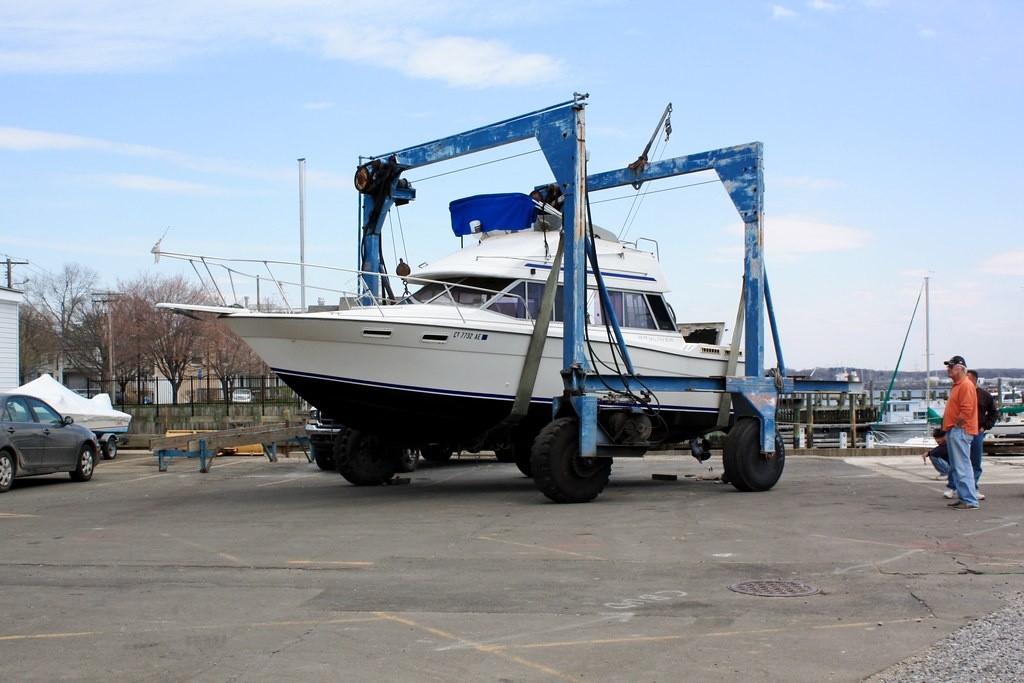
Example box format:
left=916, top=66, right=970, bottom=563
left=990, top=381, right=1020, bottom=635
left=872, top=274, right=939, bottom=448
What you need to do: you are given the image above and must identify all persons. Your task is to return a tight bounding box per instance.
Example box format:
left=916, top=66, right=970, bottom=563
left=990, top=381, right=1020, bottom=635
left=922, top=356, right=999, bottom=511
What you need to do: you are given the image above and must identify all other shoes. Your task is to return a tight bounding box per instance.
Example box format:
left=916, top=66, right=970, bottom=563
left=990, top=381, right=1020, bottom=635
left=942, top=486, right=958, bottom=499
left=936, top=473, right=949, bottom=481
left=946, top=501, right=979, bottom=510
left=975, top=490, right=985, bottom=500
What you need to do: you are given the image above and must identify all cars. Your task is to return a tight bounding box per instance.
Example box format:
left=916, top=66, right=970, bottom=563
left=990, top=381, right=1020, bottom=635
left=1, top=392, right=101, bottom=494
left=232, top=388, right=257, bottom=403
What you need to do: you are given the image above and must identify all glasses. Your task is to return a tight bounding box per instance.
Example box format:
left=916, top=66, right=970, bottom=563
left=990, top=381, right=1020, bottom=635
left=947, top=364, right=959, bottom=369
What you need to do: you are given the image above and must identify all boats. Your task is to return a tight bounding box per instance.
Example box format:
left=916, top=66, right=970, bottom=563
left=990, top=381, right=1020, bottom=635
left=155, top=193, right=750, bottom=448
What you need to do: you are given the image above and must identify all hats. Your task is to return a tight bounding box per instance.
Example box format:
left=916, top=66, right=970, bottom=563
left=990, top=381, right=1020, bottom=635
left=943, top=356, right=967, bottom=368
